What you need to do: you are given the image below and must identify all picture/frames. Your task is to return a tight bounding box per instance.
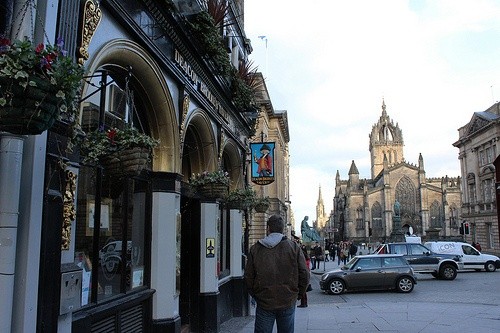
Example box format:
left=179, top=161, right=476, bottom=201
left=130, top=266, right=144, bottom=289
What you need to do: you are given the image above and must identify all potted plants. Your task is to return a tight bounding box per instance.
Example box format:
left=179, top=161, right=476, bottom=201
left=186, top=170, right=234, bottom=199
left=254, top=196, right=271, bottom=213
left=220, top=186, right=256, bottom=209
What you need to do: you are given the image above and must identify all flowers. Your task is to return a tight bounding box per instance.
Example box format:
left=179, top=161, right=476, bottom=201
left=65, top=127, right=161, bottom=165
left=0, top=36, right=86, bottom=121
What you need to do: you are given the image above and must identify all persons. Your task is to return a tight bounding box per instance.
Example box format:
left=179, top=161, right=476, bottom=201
left=243, top=214, right=311, bottom=333
left=394, top=199, right=402, bottom=217
left=301, top=215, right=321, bottom=242
left=301, top=238, right=482, bottom=270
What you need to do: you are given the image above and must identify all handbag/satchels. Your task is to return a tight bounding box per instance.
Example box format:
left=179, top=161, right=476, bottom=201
left=340, top=253, right=344, bottom=258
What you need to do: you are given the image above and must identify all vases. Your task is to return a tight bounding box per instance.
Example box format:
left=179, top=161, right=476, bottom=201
left=0, top=75, right=66, bottom=135
left=100, top=140, right=152, bottom=176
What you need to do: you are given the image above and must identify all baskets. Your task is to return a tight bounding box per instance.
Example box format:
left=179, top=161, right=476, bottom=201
left=98, top=87, right=152, bottom=179
left=224, top=170, right=254, bottom=210
left=0, top=0, right=72, bottom=136
left=196, top=151, right=227, bottom=201
left=255, top=203, right=269, bottom=213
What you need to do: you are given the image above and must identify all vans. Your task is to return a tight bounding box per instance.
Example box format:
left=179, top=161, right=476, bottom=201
left=424, top=242, right=500, bottom=272
left=99, top=241, right=132, bottom=266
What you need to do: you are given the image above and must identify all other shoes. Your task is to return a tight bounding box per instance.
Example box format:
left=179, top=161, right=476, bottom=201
left=306, top=284, right=312, bottom=292
left=297, top=305, right=308, bottom=307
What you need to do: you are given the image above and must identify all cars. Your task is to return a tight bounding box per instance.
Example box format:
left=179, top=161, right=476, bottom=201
left=319, top=254, right=418, bottom=294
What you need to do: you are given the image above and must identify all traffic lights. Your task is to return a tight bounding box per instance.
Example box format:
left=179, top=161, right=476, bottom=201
left=464, top=223, right=470, bottom=235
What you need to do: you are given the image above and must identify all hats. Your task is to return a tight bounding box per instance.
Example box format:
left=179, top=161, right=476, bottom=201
left=260, top=145, right=271, bottom=151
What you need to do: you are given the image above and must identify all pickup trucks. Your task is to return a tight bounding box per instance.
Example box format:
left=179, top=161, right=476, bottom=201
left=353, top=243, right=465, bottom=281
